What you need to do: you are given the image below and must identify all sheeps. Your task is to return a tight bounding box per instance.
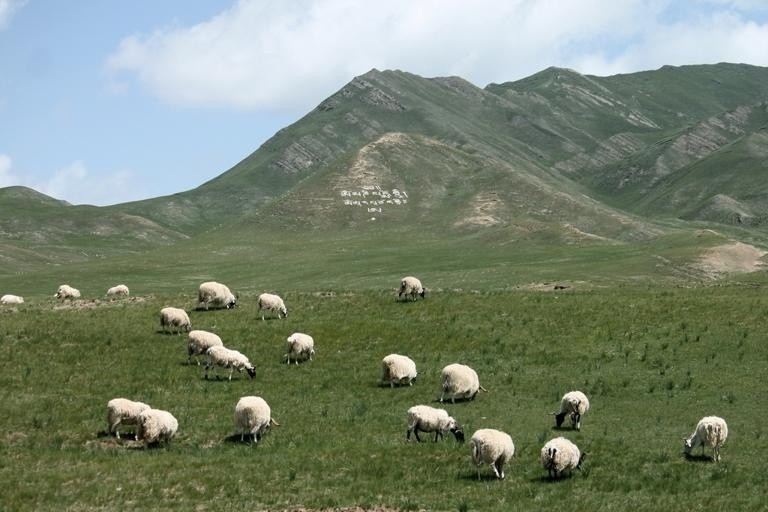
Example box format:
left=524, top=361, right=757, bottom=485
left=0, top=292, right=25, bottom=306
left=398, top=275, right=427, bottom=302
left=104, top=284, right=129, bottom=301
left=283, top=332, right=315, bottom=365
left=55, top=284, right=81, bottom=302
left=540, top=436, right=587, bottom=478
left=195, top=281, right=237, bottom=310
left=381, top=353, right=488, bottom=443
left=232, top=393, right=279, bottom=442
left=553, top=389, right=591, bottom=430
left=468, top=428, right=515, bottom=480
left=684, top=414, right=729, bottom=462
left=105, top=396, right=179, bottom=450
left=160, top=306, right=257, bottom=382
left=255, top=293, right=288, bottom=321
